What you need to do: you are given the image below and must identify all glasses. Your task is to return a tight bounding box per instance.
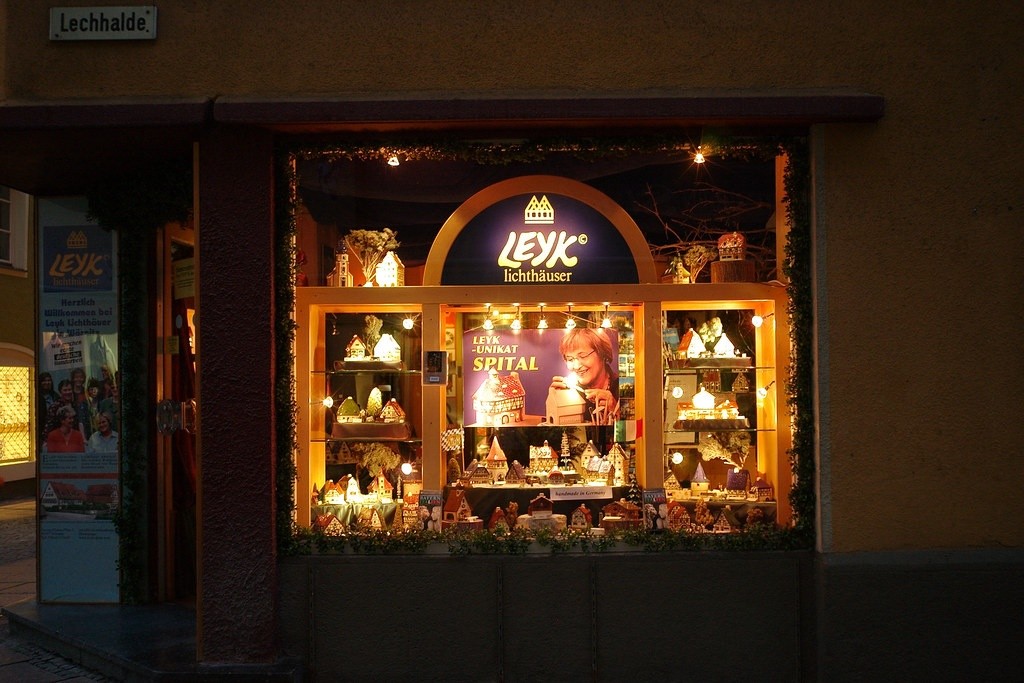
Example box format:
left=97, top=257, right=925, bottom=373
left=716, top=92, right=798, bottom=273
left=564, top=350, right=596, bottom=364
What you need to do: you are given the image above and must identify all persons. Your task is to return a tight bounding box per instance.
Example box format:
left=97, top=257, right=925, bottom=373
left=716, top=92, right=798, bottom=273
left=547, top=328, right=619, bottom=425
left=38, top=363, right=120, bottom=453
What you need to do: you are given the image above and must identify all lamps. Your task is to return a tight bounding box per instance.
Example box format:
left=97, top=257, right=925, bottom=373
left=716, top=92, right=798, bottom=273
left=386, top=148, right=401, bottom=167
left=401, top=312, right=421, bottom=330
left=663, top=449, right=684, bottom=466
left=481, top=303, right=614, bottom=334
left=692, top=144, right=708, bottom=165
left=751, top=310, right=776, bottom=327
left=400, top=460, right=422, bottom=476
left=756, top=379, right=777, bottom=400
left=310, top=396, right=334, bottom=410
left=662, top=386, right=684, bottom=400
